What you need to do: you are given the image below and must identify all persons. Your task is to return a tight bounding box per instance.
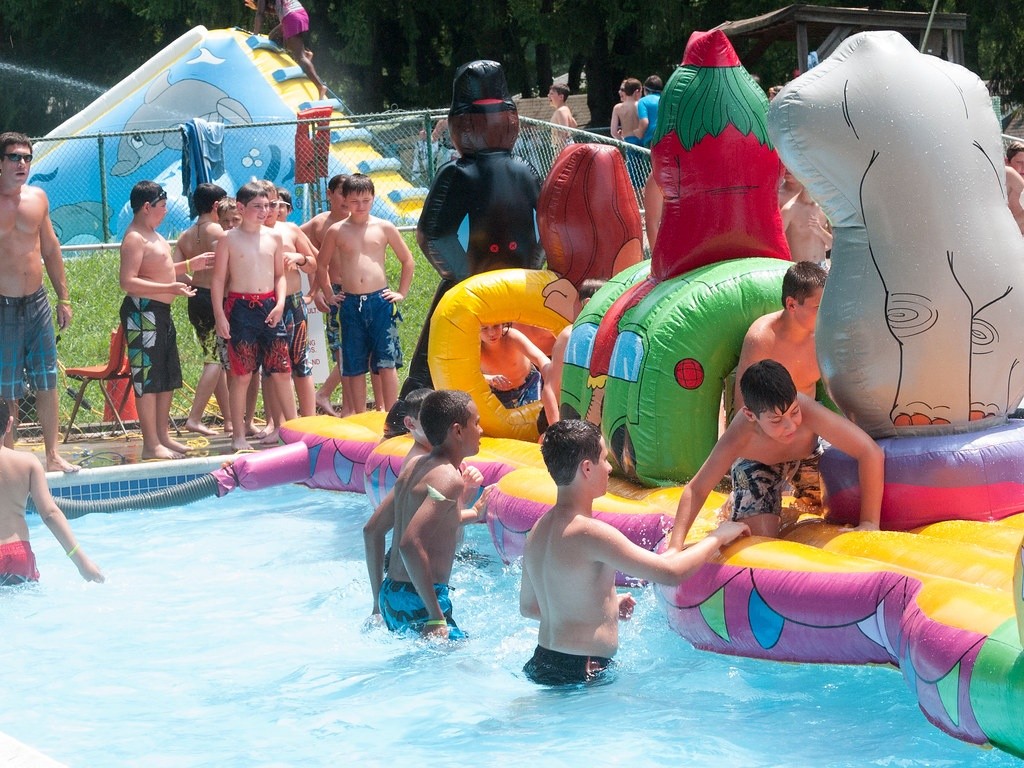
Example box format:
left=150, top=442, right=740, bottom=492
left=171, top=179, right=317, bottom=451
left=120, top=180, right=216, bottom=461
left=1004, top=140, right=1024, bottom=237
left=778, top=168, right=833, bottom=273
left=0, top=394, right=105, bottom=585
left=0, top=132, right=82, bottom=474
left=298, top=172, right=415, bottom=418
left=733, top=260, right=828, bottom=496
left=514, top=120, right=544, bottom=186
left=384, top=388, right=492, bottom=573
left=254, top=0, right=326, bottom=101
left=537, top=279, right=610, bottom=434
left=364, top=388, right=483, bottom=641
left=610, top=75, right=662, bottom=230
left=548, top=82, right=578, bottom=164
left=519, top=419, right=752, bottom=685
left=658, top=355, right=884, bottom=559
left=481, top=321, right=551, bottom=409
left=418, top=119, right=461, bottom=171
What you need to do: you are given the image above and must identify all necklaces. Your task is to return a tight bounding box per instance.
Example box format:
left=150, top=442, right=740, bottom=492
left=194, top=221, right=209, bottom=243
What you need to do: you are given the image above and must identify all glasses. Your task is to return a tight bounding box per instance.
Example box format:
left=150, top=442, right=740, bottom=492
left=279, top=201, right=291, bottom=208
left=620, top=87, right=625, bottom=92
left=270, top=200, right=280, bottom=208
left=2, top=153, right=33, bottom=162
left=151, top=192, right=167, bottom=205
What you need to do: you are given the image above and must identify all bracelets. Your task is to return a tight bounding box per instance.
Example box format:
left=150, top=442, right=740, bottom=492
left=59, top=300, right=71, bottom=305
left=186, top=259, right=191, bottom=274
left=296, top=252, right=307, bottom=266
left=425, top=620, right=447, bottom=627
left=65, top=544, right=80, bottom=558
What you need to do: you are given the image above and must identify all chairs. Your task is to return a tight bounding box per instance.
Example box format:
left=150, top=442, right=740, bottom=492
left=61, top=320, right=182, bottom=444
